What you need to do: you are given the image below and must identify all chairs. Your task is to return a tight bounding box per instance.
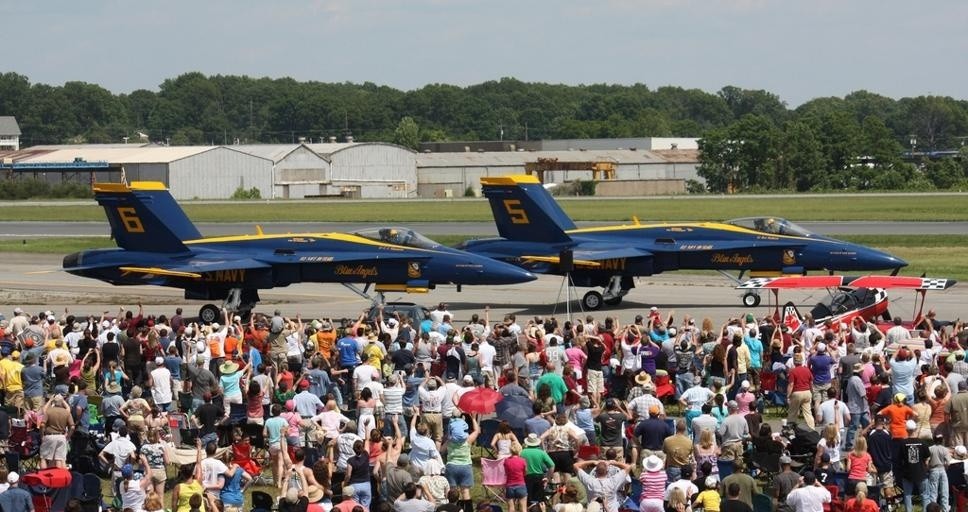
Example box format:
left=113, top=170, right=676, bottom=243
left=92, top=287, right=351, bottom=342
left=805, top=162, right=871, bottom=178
left=751, top=365, right=789, bottom=417
left=749, top=434, right=813, bottom=489
left=167, top=413, right=189, bottom=448
left=480, top=458, right=506, bottom=503
left=476, top=426, right=498, bottom=459
left=8, top=418, right=41, bottom=473
left=241, top=450, right=270, bottom=486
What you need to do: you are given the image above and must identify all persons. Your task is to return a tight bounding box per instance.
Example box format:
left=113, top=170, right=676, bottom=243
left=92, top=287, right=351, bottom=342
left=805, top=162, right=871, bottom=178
left=0, top=306, right=968, bottom=511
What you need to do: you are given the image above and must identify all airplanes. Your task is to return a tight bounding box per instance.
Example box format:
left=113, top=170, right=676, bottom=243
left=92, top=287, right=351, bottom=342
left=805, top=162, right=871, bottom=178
left=730, top=274, right=958, bottom=339
left=24, top=180, right=537, bottom=324
left=447, top=174, right=907, bottom=310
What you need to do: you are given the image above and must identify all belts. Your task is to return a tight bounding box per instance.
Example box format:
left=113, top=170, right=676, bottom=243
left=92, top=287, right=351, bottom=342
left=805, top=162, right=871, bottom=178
left=46, top=433, right=65, bottom=435
left=422, top=411, right=441, bottom=413
left=386, top=413, right=401, bottom=415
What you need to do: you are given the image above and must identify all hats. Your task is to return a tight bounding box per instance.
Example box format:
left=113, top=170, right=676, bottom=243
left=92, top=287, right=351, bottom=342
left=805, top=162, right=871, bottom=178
left=106, top=382, right=122, bottom=393
left=155, top=316, right=241, bottom=373
left=635, top=371, right=651, bottom=384
left=693, top=376, right=749, bottom=408
left=311, top=319, right=332, bottom=332
left=669, top=328, right=676, bottom=336
left=817, top=343, right=825, bottom=352
left=286, top=486, right=354, bottom=504
left=524, top=433, right=541, bottom=446
left=854, top=364, right=863, bottom=372
left=649, top=406, right=661, bottom=416
left=299, top=380, right=310, bottom=389
left=367, top=318, right=473, bottom=388
left=122, top=463, right=132, bottom=477
left=642, top=455, right=664, bottom=472
left=895, top=393, right=917, bottom=431
left=705, top=476, right=716, bottom=488
left=2, top=308, right=121, bottom=366
left=286, top=400, right=294, bottom=410
left=779, top=453, right=830, bottom=484
left=772, top=340, right=781, bottom=347
